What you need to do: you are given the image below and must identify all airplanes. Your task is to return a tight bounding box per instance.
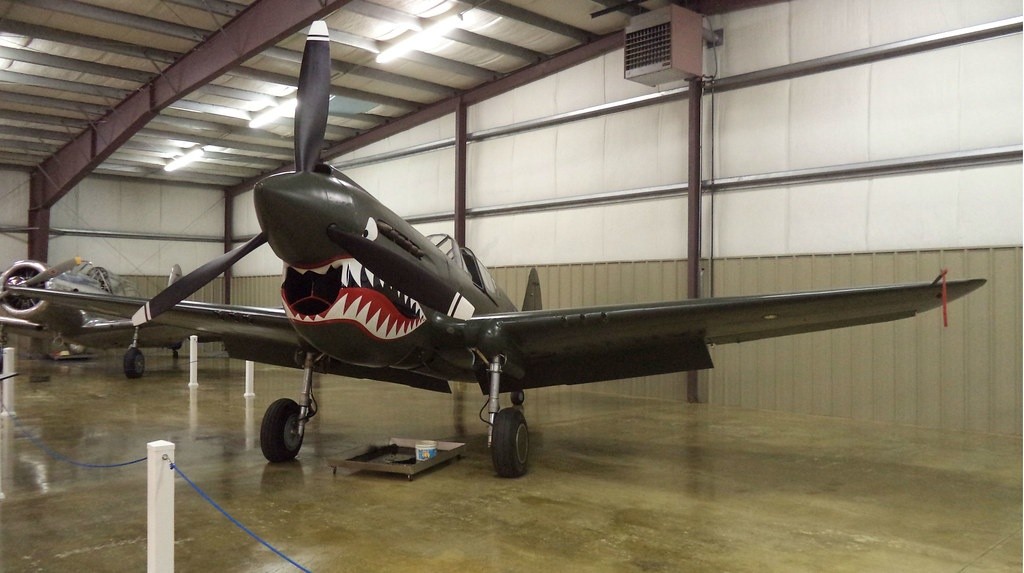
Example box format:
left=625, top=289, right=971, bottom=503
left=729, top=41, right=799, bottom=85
left=3, top=20, right=989, bottom=477
left=0, top=257, right=286, bottom=378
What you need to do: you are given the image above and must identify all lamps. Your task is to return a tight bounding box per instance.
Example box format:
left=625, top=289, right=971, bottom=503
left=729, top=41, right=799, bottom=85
left=163, top=4, right=475, bottom=176
